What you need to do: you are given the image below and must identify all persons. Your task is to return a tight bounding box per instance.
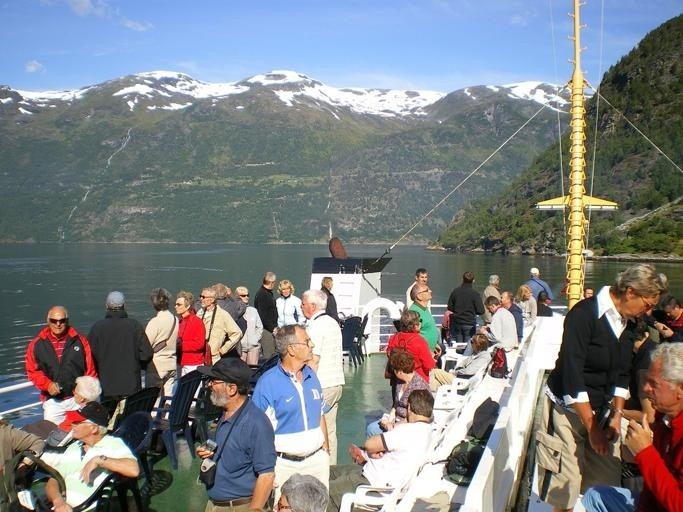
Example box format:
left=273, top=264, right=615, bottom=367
left=537, top=264, right=683, bottom=512
left=0, top=264, right=553, bottom=512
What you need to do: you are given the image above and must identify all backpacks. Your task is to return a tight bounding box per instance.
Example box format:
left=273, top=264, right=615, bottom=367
left=466, top=396, right=501, bottom=441
left=488, top=344, right=513, bottom=379
left=442, top=438, right=485, bottom=487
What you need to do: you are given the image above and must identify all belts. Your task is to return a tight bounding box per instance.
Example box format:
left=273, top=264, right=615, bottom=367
left=273, top=446, right=322, bottom=464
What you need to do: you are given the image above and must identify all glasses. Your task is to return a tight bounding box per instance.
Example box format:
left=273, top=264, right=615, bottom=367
left=46, top=317, right=67, bottom=324
left=239, top=294, right=249, bottom=298
left=173, top=302, right=188, bottom=306
left=208, top=377, right=224, bottom=384
left=417, top=287, right=431, bottom=294
left=276, top=501, right=295, bottom=509
left=640, top=296, right=659, bottom=312
left=290, top=338, right=311, bottom=348
left=417, top=318, right=422, bottom=323
left=199, top=294, right=213, bottom=299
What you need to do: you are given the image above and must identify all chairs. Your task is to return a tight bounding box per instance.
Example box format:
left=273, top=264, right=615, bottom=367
left=110, top=411, right=153, bottom=486
left=340, top=342, right=495, bottom=512
left=247, top=353, right=279, bottom=399
left=187, top=380, right=225, bottom=443
left=72, top=472, right=142, bottom=512
left=151, top=370, right=202, bottom=470
left=353, top=313, right=368, bottom=362
left=338, top=317, right=362, bottom=367
left=113, top=386, right=161, bottom=483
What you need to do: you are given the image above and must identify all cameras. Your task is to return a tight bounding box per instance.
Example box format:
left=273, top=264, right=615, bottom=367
left=199, top=438, right=217, bottom=452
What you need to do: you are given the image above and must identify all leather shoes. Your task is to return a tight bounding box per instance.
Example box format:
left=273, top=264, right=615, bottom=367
left=206, top=496, right=251, bottom=507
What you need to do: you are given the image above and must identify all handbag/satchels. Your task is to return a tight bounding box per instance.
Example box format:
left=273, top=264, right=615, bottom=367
left=198, top=457, right=217, bottom=489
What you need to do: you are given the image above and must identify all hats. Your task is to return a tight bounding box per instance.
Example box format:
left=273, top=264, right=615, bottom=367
left=530, top=267, right=540, bottom=274
left=63, top=400, right=111, bottom=427
left=196, top=357, right=253, bottom=384
left=104, top=289, right=127, bottom=305
